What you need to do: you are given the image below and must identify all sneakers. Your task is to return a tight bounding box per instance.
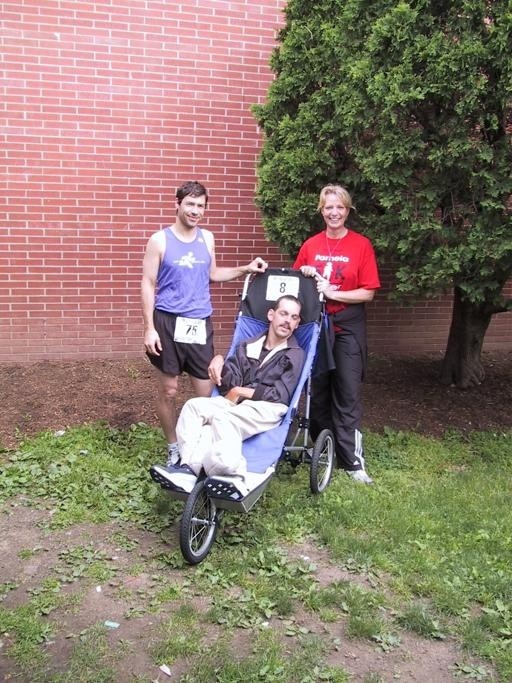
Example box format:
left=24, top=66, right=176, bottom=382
left=204, top=476, right=246, bottom=500
left=166, top=447, right=180, bottom=467
left=346, top=469, right=373, bottom=484
left=149, top=464, right=198, bottom=493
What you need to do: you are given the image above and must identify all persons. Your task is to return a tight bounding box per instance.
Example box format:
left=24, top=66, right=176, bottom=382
left=138, top=180, right=270, bottom=464
left=291, top=184, right=380, bottom=482
left=147, top=294, right=307, bottom=500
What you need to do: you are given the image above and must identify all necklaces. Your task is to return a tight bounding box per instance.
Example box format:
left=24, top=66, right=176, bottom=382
left=325, top=229, right=345, bottom=258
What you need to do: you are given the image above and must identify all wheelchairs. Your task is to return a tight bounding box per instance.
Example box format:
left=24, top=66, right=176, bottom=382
left=162, top=266, right=336, bottom=563
